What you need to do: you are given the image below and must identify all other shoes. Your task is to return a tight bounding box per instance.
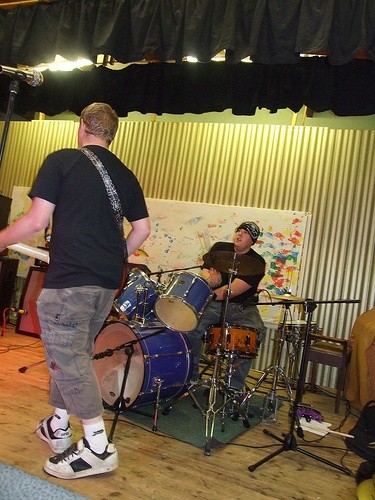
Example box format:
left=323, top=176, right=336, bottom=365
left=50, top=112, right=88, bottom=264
left=228, top=386, right=243, bottom=403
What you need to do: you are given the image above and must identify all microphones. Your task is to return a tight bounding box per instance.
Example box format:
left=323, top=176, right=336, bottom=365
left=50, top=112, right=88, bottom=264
left=235, top=289, right=263, bottom=314
left=0, top=64, right=43, bottom=87
left=8, top=307, right=28, bottom=316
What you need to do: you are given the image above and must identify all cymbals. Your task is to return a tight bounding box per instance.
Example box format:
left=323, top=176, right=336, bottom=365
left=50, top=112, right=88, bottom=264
left=262, top=292, right=303, bottom=301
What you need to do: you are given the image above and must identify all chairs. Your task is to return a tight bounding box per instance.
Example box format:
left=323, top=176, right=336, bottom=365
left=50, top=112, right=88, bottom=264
left=302, top=333, right=352, bottom=412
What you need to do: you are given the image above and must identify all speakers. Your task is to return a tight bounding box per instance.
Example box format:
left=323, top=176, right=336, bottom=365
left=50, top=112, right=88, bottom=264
left=15, top=266, right=49, bottom=339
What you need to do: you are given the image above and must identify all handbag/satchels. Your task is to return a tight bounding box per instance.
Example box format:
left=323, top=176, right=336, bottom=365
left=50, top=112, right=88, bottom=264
left=288, top=403, right=323, bottom=423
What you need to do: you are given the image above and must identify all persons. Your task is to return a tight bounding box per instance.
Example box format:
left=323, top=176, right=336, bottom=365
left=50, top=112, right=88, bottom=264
left=184, top=222, right=267, bottom=404
left=0, top=103, right=149, bottom=479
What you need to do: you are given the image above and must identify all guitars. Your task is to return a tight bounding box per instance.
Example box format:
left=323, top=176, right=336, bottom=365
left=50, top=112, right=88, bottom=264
left=7, top=243, right=127, bottom=298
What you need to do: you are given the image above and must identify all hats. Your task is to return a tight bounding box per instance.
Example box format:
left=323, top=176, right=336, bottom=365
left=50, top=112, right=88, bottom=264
left=236, top=221, right=260, bottom=245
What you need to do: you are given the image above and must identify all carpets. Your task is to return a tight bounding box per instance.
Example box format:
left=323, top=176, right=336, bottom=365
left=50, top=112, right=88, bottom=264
left=120, top=380, right=283, bottom=451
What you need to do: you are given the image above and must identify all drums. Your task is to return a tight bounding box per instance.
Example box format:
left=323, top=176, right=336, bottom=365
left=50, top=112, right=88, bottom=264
left=278, top=319, right=318, bottom=343
left=114, top=268, right=161, bottom=321
left=154, top=271, right=215, bottom=333
left=92, top=321, right=193, bottom=410
left=205, top=324, right=259, bottom=359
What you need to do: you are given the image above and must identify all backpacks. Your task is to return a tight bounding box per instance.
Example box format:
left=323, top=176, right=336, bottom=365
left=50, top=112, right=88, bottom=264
left=344, top=400, right=375, bottom=463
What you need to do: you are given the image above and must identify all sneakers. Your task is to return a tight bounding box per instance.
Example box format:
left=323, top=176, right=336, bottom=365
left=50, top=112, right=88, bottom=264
left=35, top=414, right=73, bottom=454
left=44, top=436, right=120, bottom=480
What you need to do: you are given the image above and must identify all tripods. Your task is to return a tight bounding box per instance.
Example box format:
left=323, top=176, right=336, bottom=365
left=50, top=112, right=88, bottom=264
left=89, top=266, right=361, bottom=476
